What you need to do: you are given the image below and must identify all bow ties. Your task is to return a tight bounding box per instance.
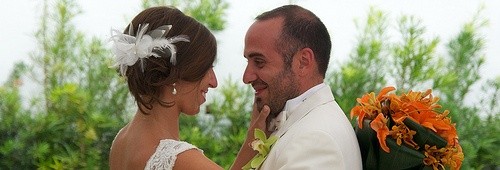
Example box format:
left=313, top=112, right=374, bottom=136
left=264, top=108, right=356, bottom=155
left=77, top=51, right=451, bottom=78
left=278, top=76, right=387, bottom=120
left=265, top=110, right=287, bottom=132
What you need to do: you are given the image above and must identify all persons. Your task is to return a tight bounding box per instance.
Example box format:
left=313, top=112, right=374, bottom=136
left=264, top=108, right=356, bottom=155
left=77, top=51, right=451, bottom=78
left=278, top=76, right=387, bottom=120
left=106, top=3, right=272, bottom=170
left=242, top=2, right=363, bottom=170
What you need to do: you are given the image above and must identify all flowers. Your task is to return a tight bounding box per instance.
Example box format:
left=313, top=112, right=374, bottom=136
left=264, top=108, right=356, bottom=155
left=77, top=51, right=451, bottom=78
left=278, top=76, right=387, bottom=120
left=348, top=85, right=464, bottom=170
left=241, top=129, right=279, bottom=169
